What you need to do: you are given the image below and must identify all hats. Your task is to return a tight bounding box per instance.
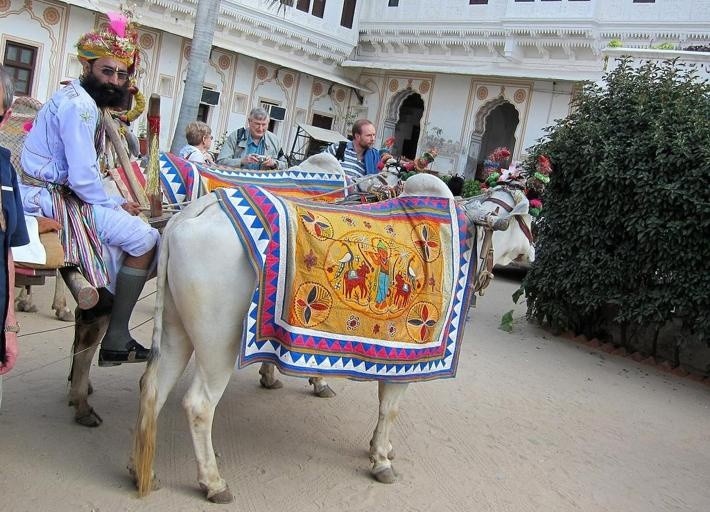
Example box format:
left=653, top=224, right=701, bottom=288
left=76, top=29, right=136, bottom=69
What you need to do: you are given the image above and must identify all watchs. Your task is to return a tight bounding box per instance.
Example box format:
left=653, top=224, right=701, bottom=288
left=271, top=160, right=279, bottom=169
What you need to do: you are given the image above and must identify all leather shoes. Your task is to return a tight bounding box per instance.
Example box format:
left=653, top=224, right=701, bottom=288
left=98, top=339, right=151, bottom=367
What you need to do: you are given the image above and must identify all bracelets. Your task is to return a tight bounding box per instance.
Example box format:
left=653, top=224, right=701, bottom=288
left=3, top=321, right=22, bottom=334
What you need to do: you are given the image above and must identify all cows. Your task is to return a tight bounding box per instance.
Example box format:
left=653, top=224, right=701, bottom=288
left=65, top=136, right=441, bottom=429
left=125, top=147, right=553, bottom=505
left=0, top=79, right=148, bottom=324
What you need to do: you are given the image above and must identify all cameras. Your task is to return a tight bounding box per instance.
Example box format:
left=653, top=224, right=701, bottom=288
left=255, top=154, right=266, bottom=165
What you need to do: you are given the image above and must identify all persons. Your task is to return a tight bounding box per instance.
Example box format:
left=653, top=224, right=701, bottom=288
left=99, top=84, right=146, bottom=170
left=0, top=65, right=31, bottom=376
left=216, top=104, right=289, bottom=171
left=318, top=119, right=381, bottom=184
left=179, top=121, right=215, bottom=164
left=17, top=31, right=160, bottom=367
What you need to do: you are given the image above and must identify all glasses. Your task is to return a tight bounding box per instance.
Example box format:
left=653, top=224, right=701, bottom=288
left=93, top=65, right=128, bottom=79
left=0, top=109, right=7, bottom=123
